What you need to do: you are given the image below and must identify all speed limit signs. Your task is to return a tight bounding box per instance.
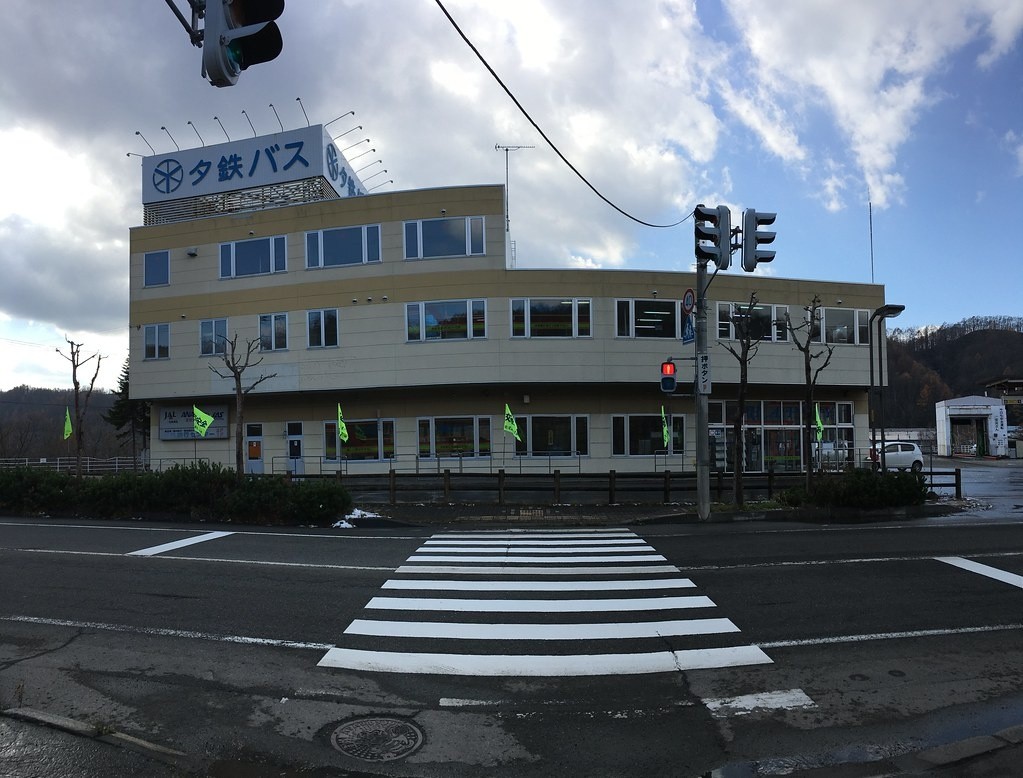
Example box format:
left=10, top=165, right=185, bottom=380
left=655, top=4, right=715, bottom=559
left=682, top=288, right=695, bottom=315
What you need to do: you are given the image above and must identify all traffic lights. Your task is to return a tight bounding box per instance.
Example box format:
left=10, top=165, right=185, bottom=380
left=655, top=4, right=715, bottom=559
left=741, top=207, right=777, bottom=272
left=661, top=362, right=677, bottom=393
left=695, top=205, right=731, bottom=271
left=202, top=0, right=285, bottom=88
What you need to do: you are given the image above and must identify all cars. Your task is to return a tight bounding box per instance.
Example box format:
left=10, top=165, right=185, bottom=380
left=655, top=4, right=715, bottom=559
left=969, top=444, right=977, bottom=455
left=866, top=441, right=924, bottom=473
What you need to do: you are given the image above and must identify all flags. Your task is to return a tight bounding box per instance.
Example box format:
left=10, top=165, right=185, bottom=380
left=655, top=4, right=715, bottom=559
left=504, top=404, right=523, bottom=443
left=661, top=405, right=671, bottom=448
left=815, top=403, right=824, bottom=442
left=337, top=402, right=349, bottom=442
left=193, top=406, right=214, bottom=436
left=64, top=407, right=73, bottom=440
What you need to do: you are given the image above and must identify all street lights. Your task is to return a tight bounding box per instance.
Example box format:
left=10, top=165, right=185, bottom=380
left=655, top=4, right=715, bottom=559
left=869, top=304, right=905, bottom=473
